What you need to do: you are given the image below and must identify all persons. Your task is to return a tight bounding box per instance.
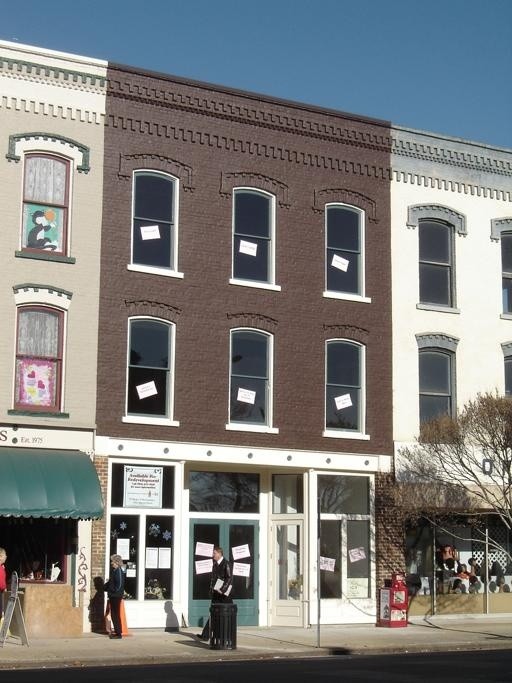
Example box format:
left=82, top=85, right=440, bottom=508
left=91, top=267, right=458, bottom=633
left=196, top=545, right=233, bottom=641
left=0, top=547, right=8, bottom=622
left=104, top=554, right=126, bottom=639
left=444, top=557, right=504, bottom=593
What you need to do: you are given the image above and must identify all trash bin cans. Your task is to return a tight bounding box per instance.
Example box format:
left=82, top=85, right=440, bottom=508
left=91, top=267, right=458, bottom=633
left=208, top=603, right=238, bottom=650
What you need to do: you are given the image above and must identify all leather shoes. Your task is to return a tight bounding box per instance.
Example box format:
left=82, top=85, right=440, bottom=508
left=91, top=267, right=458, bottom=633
left=196, top=633, right=208, bottom=640
left=110, top=633, right=122, bottom=638
